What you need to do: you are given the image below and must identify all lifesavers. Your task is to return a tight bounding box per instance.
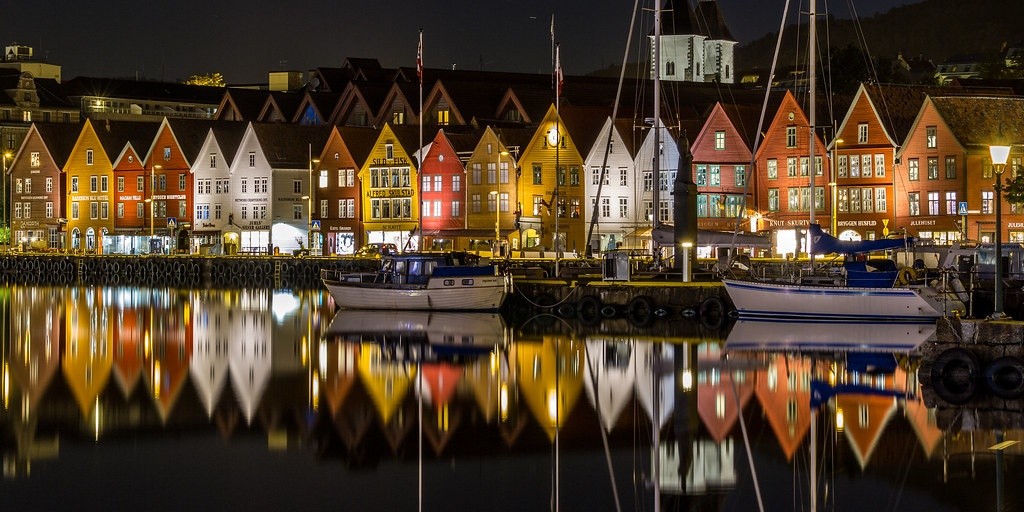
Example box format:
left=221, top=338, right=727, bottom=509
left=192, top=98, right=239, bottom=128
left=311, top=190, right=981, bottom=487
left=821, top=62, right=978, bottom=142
left=931, top=377, right=983, bottom=405
left=532, top=312, right=726, bottom=330
left=0, top=258, right=376, bottom=274
left=3, top=272, right=324, bottom=289
left=898, top=267, right=916, bottom=286
left=983, top=378, right=1024, bottom=399
left=534, top=295, right=743, bottom=315
left=931, top=348, right=982, bottom=377
left=982, top=356, right=1024, bottom=378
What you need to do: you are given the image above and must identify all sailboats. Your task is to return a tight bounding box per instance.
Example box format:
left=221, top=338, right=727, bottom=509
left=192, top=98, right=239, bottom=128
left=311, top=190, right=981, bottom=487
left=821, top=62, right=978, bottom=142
left=718, top=1, right=975, bottom=318
left=723, top=314, right=937, bottom=512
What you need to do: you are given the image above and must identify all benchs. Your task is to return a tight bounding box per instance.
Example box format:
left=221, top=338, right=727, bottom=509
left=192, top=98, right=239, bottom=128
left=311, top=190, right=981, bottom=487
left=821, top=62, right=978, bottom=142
left=561, top=268, right=602, bottom=280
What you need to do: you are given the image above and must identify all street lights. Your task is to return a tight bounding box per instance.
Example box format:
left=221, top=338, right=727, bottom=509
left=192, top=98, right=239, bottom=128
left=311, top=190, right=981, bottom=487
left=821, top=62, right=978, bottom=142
left=834, top=139, right=844, bottom=240
left=2, top=152, right=12, bottom=253
left=149, top=164, right=162, bottom=253
left=307, top=143, right=320, bottom=257
left=991, top=124, right=1013, bottom=313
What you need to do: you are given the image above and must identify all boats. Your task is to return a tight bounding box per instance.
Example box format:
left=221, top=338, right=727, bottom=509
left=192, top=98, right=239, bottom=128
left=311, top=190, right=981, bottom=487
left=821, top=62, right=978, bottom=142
left=319, top=26, right=510, bottom=311
left=323, top=306, right=510, bottom=512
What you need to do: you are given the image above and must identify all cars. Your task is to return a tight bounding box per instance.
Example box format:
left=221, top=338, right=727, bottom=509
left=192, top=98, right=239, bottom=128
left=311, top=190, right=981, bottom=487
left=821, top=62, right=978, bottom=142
left=354, top=242, right=398, bottom=257
